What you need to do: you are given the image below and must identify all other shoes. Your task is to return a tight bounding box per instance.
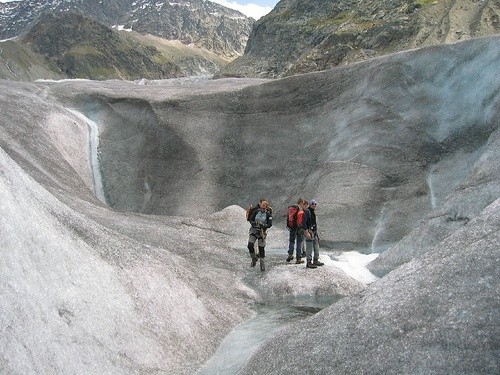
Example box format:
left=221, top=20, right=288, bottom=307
left=260, top=261, right=265, bottom=271
left=287, top=255, right=293, bottom=262
left=251, top=257, right=258, bottom=267
left=296, top=258, right=304, bottom=264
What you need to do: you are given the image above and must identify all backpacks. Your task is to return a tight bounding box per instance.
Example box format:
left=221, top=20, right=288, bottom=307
left=287, top=204, right=300, bottom=228
left=296, top=208, right=311, bottom=227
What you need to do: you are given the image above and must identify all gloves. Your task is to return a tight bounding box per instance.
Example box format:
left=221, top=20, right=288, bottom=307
left=259, top=223, right=267, bottom=228
left=251, top=221, right=256, bottom=226
left=304, top=233, right=311, bottom=240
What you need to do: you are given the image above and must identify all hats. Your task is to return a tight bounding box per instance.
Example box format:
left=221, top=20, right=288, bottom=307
left=309, top=199, right=316, bottom=205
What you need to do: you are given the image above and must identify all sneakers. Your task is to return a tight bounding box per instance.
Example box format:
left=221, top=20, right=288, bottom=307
left=313, top=260, right=324, bottom=266
left=307, top=262, right=317, bottom=268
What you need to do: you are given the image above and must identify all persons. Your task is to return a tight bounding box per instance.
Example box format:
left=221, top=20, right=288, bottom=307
left=247, top=198, right=273, bottom=272
left=286, top=197, right=308, bottom=264
left=302, top=199, right=325, bottom=268
